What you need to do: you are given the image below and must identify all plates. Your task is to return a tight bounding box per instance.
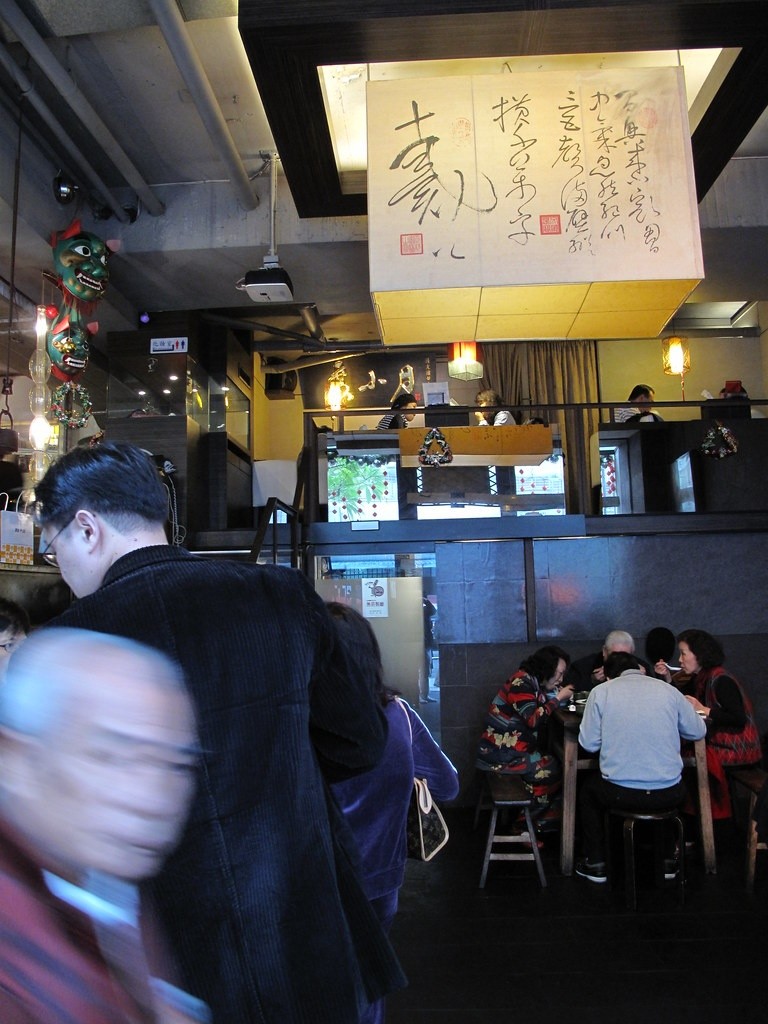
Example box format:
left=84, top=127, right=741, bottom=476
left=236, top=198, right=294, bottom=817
left=575, top=699, right=588, bottom=704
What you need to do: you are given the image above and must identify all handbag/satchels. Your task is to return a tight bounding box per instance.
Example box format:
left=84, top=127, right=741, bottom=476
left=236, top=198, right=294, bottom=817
left=395, top=697, right=450, bottom=864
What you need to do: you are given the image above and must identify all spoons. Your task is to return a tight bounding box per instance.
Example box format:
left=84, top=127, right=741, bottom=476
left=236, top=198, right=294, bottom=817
left=664, top=661, right=683, bottom=671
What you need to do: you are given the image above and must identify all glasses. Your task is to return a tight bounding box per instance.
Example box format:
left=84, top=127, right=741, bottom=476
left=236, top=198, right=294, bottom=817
left=41, top=511, right=96, bottom=568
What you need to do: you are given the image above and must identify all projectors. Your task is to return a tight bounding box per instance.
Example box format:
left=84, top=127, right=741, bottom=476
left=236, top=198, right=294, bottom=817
left=245, top=268, right=294, bottom=303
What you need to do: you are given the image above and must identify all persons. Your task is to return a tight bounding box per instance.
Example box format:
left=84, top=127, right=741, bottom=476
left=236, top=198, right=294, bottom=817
left=719, top=386, right=767, bottom=418
left=475, top=629, right=761, bottom=883
left=614, top=384, right=664, bottom=422
left=474, top=389, right=517, bottom=426
left=376, top=394, right=417, bottom=430
left=0, top=441, right=459, bottom=1024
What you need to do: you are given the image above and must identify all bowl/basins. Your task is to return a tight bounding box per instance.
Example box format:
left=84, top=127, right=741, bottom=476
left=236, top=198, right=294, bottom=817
left=546, top=692, right=567, bottom=706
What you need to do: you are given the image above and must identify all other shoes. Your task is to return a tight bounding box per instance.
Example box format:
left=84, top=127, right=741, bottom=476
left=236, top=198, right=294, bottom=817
left=510, top=828, right=545, bottom=851
left=672, top=844, right=693, bottom=869
left=575, top=857, right=607, bottom=883
left=663, top=859, right=681, bottom=879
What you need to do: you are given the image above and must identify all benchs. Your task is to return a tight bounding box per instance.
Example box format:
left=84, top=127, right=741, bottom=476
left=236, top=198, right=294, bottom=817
left=471, top=772, right=550, bottom=889
left=731, top=762, right=768, bottom=887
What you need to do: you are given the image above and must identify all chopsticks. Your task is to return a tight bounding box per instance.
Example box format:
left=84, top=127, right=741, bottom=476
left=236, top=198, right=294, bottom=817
left=555, top=684, right=578, bottom=691
left=590, top=671, right=599, bottom=673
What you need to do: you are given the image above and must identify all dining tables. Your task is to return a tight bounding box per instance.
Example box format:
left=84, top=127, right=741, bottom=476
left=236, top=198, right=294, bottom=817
left=554, top=702, right=717, bottom=878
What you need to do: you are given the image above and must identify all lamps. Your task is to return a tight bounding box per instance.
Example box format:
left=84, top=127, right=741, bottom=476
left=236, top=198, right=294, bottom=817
left=447, top=342, right=483, bottom=381
left=661, top=335, right=691, bottom=376
